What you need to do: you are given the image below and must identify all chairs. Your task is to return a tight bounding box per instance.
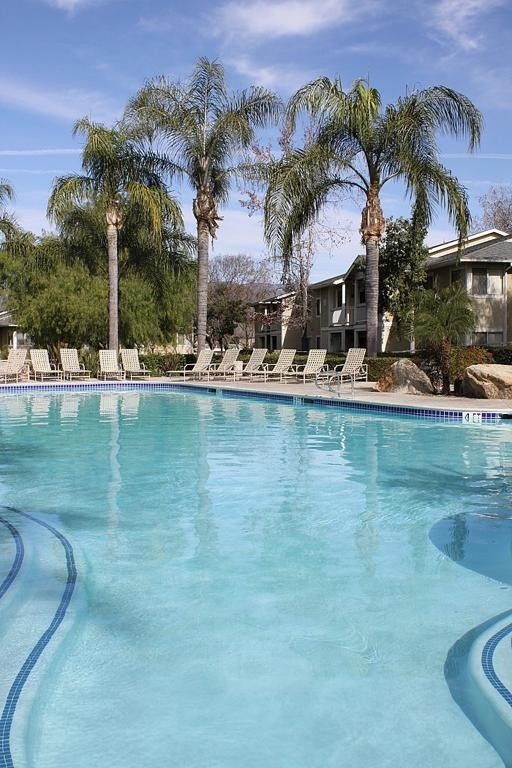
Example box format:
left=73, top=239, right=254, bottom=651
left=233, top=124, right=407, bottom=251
left=166, top=346, right=369, bottom=396
left=1, top=348, right=153, bottom=384
